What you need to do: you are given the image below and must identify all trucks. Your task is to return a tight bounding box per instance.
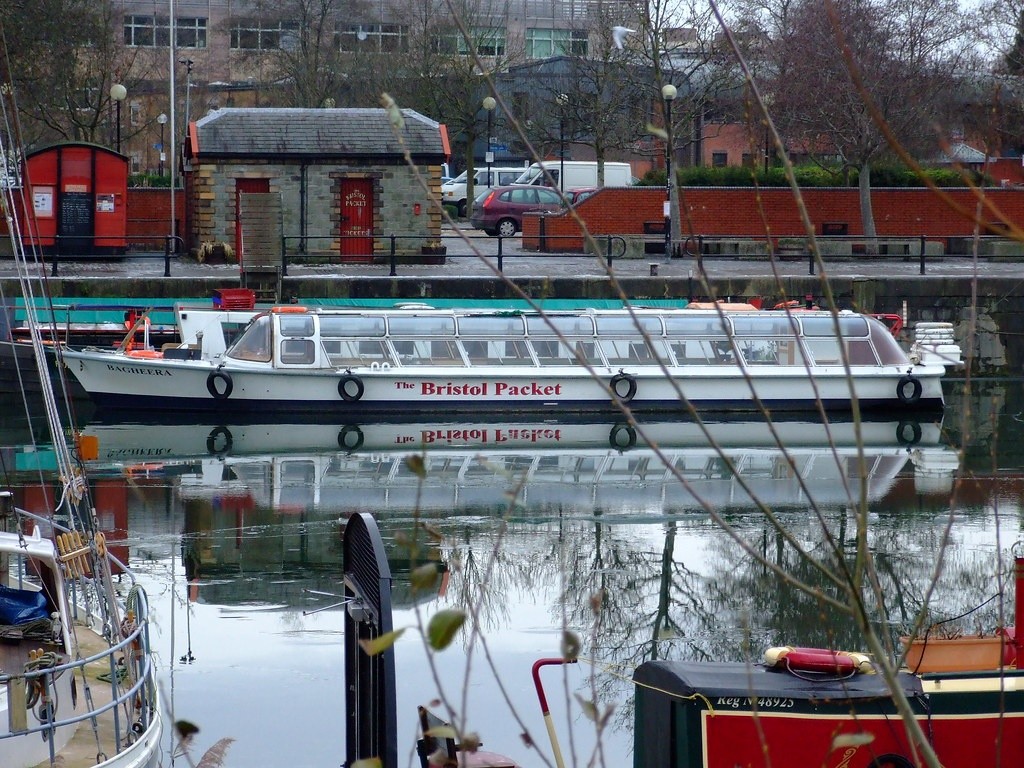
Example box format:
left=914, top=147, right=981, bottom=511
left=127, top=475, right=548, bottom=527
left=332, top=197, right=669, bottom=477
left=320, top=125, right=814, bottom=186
left=510, top=162, right=632, bottom=209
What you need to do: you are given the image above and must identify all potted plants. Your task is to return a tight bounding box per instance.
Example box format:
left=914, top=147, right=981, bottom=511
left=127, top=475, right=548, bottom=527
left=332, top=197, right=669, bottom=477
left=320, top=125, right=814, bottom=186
left=778, top=237, right=806, bottom=261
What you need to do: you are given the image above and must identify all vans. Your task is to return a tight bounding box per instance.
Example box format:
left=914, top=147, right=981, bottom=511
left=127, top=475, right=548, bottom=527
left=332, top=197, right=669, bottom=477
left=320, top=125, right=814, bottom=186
left=440, top=166, right=527, bottom=218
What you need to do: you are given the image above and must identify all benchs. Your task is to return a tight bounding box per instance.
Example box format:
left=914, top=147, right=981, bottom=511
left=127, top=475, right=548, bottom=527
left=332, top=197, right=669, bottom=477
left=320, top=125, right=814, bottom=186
left=582, top=234, right=1024, bottom=263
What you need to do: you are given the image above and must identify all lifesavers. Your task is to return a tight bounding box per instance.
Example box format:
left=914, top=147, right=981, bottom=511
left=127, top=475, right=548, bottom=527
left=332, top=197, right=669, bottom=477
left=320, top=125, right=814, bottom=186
left=896, top=375, right=923, bottom=404
left=124, top=315, right=151, bottom=332
left=609, top=373, right=638, bottom=403
left=337, top=375, right=365, bottom=402
left=272, top=306, right=309, bottom=313
left=337, top=424, right=364, bottom=453
left=609, top=423, right=638, bottom=452
left=896, top=421, right=923, bottom=445
left=775, top=300, right=799, bottom=308
left=205, top=370, right=233, bottom=400
left=206, top=427, right=234, bottom=458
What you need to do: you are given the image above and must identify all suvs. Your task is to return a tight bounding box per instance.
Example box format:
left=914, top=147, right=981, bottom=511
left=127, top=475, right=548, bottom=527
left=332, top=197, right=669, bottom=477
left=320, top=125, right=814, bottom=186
left=470, top=185, right=571, bottom=237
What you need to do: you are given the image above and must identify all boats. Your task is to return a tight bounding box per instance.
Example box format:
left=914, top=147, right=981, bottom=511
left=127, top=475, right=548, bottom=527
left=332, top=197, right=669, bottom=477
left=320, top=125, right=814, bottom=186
left=57, top=296, right=946, bottom=411
left=0, top=490, right=164, bottom=768
left=73, top=411, right=945, bottom=512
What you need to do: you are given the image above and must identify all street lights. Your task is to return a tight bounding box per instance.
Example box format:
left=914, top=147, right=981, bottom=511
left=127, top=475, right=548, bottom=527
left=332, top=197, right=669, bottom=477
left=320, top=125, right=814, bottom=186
left=482, top=96, right=496, bottom=189
left=110, top=84, right=127, bottom=154
left=662, top=84, right=678, bottom=264
left=157, top=112, right=167, bottom=177
left=555, top=94, right=569, bottom=194
